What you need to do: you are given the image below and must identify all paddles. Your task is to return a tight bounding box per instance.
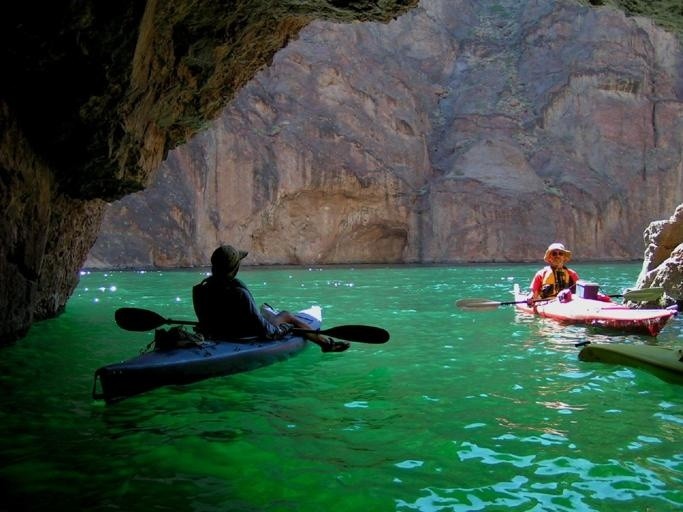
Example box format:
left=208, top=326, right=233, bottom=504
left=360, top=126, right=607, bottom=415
left=116, top=307, right=390, bottom=344
left=457, top=283, right=664, bottom=312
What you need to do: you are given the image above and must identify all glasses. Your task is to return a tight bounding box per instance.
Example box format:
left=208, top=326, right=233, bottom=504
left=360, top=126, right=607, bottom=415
left=551, top=251, right=564, bottom=256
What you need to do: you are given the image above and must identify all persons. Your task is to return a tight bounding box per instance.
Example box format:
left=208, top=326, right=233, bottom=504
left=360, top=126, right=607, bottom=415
left=527, top=243, right=578, bottom=306
left=192, top=245, right=350, bottom=352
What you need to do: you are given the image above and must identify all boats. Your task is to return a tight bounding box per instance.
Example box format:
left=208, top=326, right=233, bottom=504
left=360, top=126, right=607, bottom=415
left=511, top=279, right=677, bottom=341
left=93, top=301, right=327, bottom=400
left=572, top=338, right=682, bottom=388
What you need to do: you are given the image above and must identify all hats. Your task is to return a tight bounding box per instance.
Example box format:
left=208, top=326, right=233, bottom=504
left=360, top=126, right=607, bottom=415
left=211, top=244, right=248, bottom=273
left=544, top=242, right=572, bottom=265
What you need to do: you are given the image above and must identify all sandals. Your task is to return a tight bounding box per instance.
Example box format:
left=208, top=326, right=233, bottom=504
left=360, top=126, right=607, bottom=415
left=322, top=339, right=350, bottom=352
